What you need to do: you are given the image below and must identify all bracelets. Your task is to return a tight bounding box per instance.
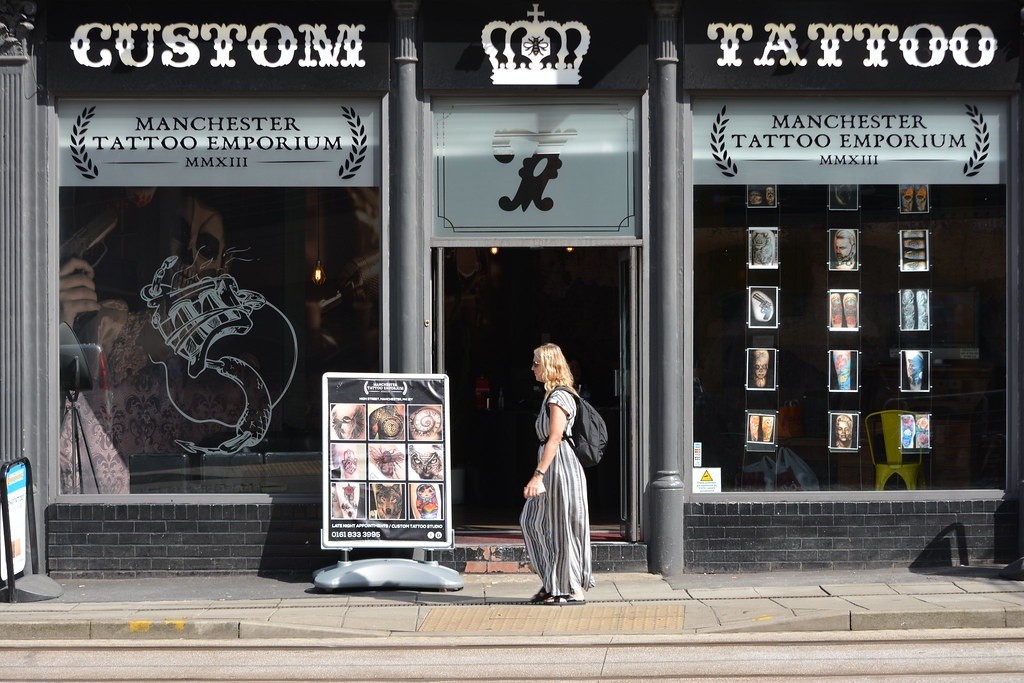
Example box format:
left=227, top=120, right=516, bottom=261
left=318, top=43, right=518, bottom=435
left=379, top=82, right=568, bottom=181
left=534, top=469, right=545, bottom=476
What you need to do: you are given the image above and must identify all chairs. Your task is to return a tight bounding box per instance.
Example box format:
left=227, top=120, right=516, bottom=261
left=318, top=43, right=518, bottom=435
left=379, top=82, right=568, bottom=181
left=865, top=410, right=926, bottom=490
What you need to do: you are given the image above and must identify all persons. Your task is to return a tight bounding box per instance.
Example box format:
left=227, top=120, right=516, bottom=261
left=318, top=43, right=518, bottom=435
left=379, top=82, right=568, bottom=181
left=518, top=344, right=595, bottom=603
left=528, top=348, right=600, bottom=474
left=60, top=192, right=269, bottom=493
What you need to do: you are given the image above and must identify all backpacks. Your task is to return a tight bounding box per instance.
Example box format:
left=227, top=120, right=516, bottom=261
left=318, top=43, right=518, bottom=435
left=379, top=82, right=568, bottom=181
left=546, top=386, right=607, bottom=467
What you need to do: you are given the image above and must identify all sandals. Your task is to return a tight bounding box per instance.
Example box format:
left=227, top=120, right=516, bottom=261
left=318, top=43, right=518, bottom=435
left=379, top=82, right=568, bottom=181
left=531, top=586, right=585, bottom=604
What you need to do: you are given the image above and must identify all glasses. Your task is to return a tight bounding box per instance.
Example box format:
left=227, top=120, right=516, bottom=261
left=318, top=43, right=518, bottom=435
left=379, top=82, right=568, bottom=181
left=533, top=361, right=541, bottom=368
